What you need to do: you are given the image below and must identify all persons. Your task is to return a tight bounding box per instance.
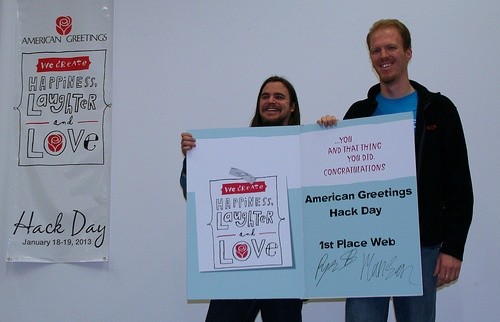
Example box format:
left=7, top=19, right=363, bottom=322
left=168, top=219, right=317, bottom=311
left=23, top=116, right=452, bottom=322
left=340, top=18, right=466, bottom=322
left=176, top=75, right=338, bottom=322
